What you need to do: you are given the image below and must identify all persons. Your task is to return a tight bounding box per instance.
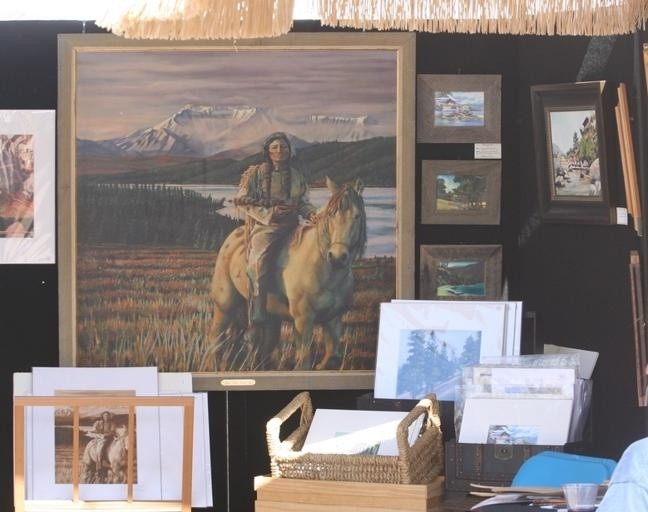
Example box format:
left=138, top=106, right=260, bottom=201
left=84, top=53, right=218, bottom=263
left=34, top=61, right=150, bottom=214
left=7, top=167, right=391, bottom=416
left=91, top=409, right=117, bottom=478
left=233, top=130, right=319, bottom=344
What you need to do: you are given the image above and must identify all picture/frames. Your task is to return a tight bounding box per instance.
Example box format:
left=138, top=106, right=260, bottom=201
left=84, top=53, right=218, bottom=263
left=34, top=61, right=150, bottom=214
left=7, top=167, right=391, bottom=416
left=457, top=354, right=594, bottom=444
left=53, top=31, right=422, bottom=396
left=528, top=78, right=620, bottom=229
left=29, top=363, right=165, bottom=500
left=417, top=158, right=506, bottom=227
left=415, top=70, right=503, bottom=148
left=416, top=242, right=507, bottom=301
left=373, top=301, right=523, bottom=400
left=0, top=105, right=61, bottom=272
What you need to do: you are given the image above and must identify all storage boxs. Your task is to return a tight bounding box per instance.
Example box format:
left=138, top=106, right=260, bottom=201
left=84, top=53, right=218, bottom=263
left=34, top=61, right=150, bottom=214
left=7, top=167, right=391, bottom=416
left=443, top=436, right=580, bottom=493
left=355, top=392, right=455, bottom=441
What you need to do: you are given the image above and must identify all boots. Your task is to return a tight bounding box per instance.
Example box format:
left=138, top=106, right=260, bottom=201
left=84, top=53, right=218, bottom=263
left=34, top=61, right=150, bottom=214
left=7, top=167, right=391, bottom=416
left=251, top=296, right=268, bottom=323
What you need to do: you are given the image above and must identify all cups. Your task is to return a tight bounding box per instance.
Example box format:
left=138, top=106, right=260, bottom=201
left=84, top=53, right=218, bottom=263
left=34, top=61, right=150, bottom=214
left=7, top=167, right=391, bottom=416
left=560, top=483, right=600, bottom=512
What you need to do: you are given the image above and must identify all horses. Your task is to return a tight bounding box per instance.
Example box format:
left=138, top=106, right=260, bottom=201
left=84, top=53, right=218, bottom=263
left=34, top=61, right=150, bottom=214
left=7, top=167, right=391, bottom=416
left=81, top=424, right=128, bottom=484
left=203, top=175, right=367, bottom=372
